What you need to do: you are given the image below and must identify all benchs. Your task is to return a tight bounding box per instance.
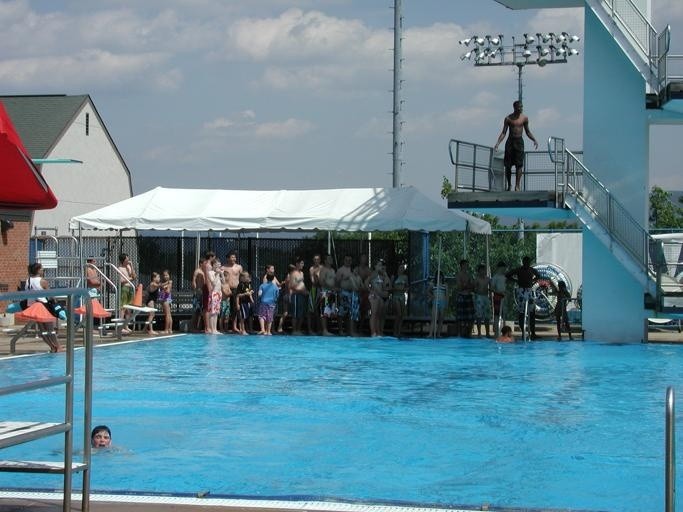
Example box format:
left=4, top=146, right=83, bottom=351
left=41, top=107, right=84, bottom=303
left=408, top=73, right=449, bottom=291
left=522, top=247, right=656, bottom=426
left=386, top=314, right=458, bottom=336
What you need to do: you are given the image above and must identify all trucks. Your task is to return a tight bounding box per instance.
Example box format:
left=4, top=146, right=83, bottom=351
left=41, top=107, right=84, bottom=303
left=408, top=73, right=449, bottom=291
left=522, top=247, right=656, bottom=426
left=533, top=229, right=681, bottom=330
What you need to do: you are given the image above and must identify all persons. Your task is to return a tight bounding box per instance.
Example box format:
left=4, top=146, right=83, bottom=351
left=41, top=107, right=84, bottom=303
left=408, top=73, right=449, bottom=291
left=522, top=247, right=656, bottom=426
left=190, top=250, right=492, bottom=336
left=144, top=271, right=174, bottom=336
left=85, top=255, right=102, bottom=302
left=489, top=256, right=575, bottom=343
left=493, top=99, right=538, bottom=192
left=115, top=251, right=138, bottom=335
left=22, top=263, right=56, bottom=311
left=76, top=423, right=133, bottom=460
left=157, top=267, right=174, bottom=334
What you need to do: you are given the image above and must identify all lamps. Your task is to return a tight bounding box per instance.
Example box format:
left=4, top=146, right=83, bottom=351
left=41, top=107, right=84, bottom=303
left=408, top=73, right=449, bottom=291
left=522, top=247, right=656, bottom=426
left=100, top=248, right=108, bottom=257
left=0, top=214, right=14, bottom=232
